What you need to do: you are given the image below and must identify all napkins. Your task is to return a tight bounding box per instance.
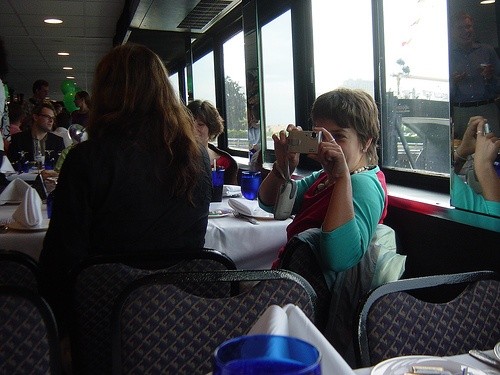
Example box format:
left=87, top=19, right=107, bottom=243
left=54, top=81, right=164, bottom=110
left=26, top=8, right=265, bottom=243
left=248, top=303, right=351, bottom=375
left=221, top=185, right=241, bottom=198
left=10, top=187, right=42, bottom=226
left=0, top=179, right=33, bottom=204
left=0, top=155, right=16, bottom=173
left=229, top=196, right=275, bottom=220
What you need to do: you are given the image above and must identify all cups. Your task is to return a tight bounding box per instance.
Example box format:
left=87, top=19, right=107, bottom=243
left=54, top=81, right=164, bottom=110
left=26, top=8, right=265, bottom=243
left=211, top=168, right=223, bottom=202
left=17, top=160, right=30, bottom=173
left=46, top=190, right=56, bottom=219
left=213, top=334, right=323, bottom=375
left=45, top=150, right=57, bottom=169
left=241, top=171, right=261, bottom=200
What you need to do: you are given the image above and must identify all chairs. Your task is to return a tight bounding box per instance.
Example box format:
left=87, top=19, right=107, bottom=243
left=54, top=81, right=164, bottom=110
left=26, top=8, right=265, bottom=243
left=0, top=251, right=500, bottom=375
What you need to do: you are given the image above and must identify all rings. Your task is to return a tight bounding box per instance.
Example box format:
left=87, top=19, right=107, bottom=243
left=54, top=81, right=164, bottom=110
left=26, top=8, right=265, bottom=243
left=329, top=139, right=335, bottom=143
left=466, top=123, right=470, bottom=126
left=476, top=130, right=485, bottom=136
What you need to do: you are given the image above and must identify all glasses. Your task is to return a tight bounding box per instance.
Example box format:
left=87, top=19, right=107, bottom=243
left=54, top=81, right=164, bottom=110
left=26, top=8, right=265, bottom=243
left=40, top=114, right=56, bottom=121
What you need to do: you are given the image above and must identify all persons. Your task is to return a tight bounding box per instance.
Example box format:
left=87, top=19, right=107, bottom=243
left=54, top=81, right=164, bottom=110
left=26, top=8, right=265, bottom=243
left=0, top=78, right=92, bottom=200
left=257, top=89, right=389, bottom=273
left=450, top=116, right=500, bottom=218
left=185, top=99, right=239, bottom=202
left=37, top=42, right=213, bottom=375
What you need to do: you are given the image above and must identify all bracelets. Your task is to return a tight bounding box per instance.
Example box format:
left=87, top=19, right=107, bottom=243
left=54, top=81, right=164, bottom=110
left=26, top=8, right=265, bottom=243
left=265, top=176, right=284, bottom=187
left=453, top=149, right=472, bottom=161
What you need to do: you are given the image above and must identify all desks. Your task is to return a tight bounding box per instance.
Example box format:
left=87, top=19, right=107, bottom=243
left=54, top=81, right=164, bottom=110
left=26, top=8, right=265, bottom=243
left=0, top=184, right=295, bottom=295
left=6, top=167, right=52, bottom=185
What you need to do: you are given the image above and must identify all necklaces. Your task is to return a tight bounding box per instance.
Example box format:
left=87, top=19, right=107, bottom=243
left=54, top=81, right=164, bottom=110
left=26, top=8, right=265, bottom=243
left=313, top=164, right=377, bottom=195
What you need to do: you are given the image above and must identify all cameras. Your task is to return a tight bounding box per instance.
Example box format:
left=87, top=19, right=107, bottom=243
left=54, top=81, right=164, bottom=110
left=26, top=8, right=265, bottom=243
left=288, top=131, right=323, bottom=154
left=483, top=123, right=492, bottom=135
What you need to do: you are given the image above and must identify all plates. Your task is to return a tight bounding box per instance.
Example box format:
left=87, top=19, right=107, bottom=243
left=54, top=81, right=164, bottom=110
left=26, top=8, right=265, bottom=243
left=6, top=218, right=51, bottom=230
left=494, top=342, right=500, bottom=361
left=236, top=203, right=279, bottom=219
left=370, top=355, right=489, bottom=375
left=222, top=185, right=242, bottom=197
left=206, top=207, right=235, bottom=218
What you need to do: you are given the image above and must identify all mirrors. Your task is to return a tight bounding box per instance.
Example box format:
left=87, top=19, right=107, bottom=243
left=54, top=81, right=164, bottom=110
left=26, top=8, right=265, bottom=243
left=447, top=0, right=500, bottom=217
